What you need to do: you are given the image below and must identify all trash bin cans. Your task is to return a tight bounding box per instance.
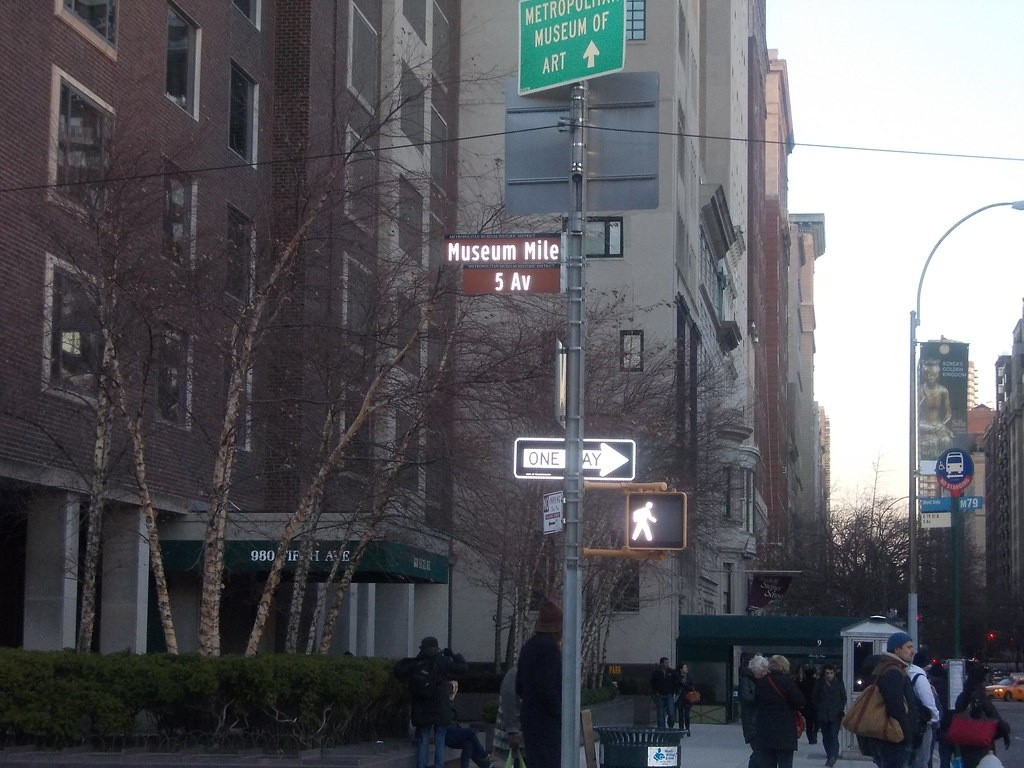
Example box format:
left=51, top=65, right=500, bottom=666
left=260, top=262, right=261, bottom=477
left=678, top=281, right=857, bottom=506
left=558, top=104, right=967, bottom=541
left=593, top=726, right=687, bottom=768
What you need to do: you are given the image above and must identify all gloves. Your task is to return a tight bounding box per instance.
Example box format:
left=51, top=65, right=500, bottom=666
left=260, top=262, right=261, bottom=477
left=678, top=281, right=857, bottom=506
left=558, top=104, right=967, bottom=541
left=1004, top=735, right=1011, bottom=750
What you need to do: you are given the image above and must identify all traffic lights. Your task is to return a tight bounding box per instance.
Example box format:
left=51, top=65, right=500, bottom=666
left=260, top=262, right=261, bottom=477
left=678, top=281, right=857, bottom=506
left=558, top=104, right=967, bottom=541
left=988, top=633, right=995, bottom=640
left=624, top=492, right=690, bottom=554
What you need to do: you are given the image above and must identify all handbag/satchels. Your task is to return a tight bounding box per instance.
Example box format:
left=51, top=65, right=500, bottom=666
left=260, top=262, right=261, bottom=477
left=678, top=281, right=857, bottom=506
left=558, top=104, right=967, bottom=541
left=842, top=663, right=909, bottom=743
left=793, top=711, right=806, bottom=737
left=945, top=706, right=998, bottom=750
left=687, top=687, right=700, bottom=703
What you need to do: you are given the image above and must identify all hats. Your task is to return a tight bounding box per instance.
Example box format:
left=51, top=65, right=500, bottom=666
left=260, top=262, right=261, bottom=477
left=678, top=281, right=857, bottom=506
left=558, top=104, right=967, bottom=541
left=888, top=632, right=913, bottom=651
left=419, top=636, right=441, bottom=650
left=912, top=652, right=930, bottom=667
left=534, top=605, right=567, bottom=634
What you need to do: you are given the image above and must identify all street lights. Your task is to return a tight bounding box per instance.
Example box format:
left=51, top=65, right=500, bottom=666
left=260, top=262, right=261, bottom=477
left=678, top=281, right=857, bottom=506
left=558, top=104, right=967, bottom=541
left=908, top=202, right=1024, bottom=653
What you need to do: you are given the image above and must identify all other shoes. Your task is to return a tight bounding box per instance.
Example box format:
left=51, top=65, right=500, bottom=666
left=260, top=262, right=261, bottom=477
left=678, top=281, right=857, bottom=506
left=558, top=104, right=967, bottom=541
left=809, top=735, right=817, bottom=744
left=825, top=757, right=836, bottom=768
left=686, top=729, right=691, bottom=737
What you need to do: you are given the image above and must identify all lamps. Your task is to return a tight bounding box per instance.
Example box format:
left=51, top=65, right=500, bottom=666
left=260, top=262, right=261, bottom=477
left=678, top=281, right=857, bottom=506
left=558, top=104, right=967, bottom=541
left=751, top=333, right=758, bottom=343
left=748, top=319, right=756, bottom=329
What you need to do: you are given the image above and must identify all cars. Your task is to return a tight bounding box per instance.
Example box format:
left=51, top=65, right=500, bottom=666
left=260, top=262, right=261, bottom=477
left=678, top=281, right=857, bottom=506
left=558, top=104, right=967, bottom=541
left=923, top=663, right=1024, bottom=702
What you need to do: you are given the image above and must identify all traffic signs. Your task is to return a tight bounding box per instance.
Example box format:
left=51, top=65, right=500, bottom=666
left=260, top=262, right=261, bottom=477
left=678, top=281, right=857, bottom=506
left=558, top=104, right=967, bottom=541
left=516, top=1, right=626, bottom=93
left=515, top=438, right=638, bottom=482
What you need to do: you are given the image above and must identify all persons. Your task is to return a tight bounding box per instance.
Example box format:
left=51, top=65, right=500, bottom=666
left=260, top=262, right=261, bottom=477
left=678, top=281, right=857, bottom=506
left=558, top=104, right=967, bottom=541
left=517, top=596, right=563, bottom=768
left=493, top=668, right=525, bottom=750
left=394, top=637, right=494, bottom=768
left=855, top=633, right=1010, bottom=768
left=801, top=669, right=820, bottom=744
left=738, top=654, right=807, bottom=768
left=650, top=657, right=695, bottom=738
left=810, top=664, right=847, bottom=768
